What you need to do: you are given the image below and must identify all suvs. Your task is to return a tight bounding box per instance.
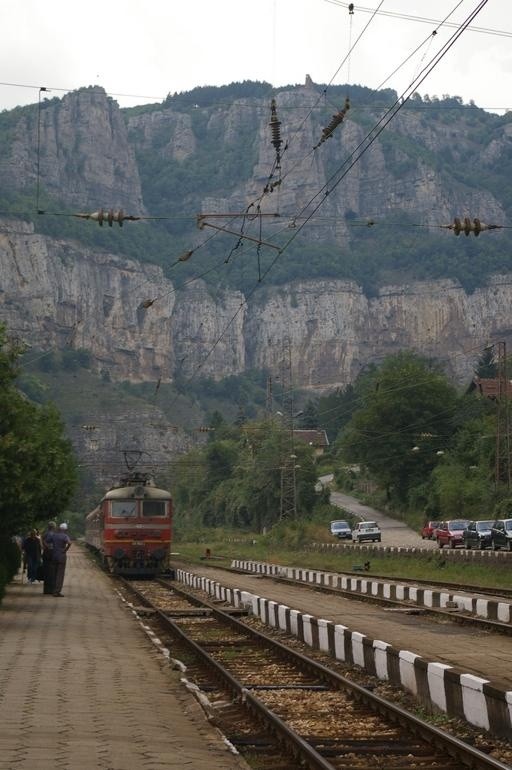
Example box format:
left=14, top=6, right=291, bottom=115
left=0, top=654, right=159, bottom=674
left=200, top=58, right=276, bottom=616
left=330, top=520, right=351, bottom=539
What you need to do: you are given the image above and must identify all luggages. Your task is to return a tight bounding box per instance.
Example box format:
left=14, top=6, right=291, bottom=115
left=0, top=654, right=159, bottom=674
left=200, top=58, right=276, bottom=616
left=36, top=564, right=45, bottom=583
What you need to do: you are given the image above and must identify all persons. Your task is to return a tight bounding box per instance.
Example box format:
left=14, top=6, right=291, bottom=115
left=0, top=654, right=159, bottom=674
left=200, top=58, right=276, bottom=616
left=46, top=522, right=72, bottom=597
left=40, top=521, right=57, bottom=593
left=19, top=527, right=41, bottom=583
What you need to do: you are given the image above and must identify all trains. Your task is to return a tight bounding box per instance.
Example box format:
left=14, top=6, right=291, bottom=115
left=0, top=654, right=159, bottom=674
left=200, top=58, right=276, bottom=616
left=85, top=485, right=172, bottom=575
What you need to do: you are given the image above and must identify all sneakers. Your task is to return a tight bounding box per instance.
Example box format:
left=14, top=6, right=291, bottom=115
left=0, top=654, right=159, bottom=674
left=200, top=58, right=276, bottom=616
left=53, top=593, right=64, bottom=597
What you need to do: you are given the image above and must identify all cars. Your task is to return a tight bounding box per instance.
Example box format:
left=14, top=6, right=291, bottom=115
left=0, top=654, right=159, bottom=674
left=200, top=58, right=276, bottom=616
left=351, top=521, right=381, bottom=543
left=422, top=519, right=512, bottom=551
